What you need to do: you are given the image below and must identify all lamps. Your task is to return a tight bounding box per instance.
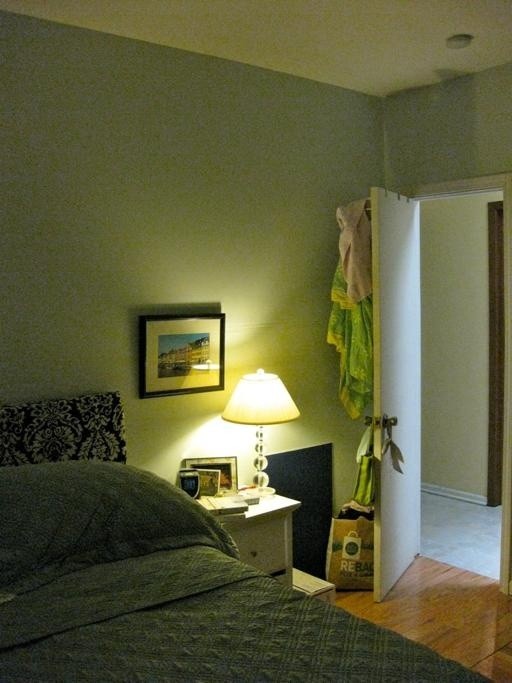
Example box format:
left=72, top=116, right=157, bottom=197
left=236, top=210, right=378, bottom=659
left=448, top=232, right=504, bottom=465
left=221, top=368, right=301, bottom=497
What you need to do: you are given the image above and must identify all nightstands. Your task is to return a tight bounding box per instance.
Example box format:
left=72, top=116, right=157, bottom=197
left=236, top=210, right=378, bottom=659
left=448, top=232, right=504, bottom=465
left=194, top=484, right=303, bottom=589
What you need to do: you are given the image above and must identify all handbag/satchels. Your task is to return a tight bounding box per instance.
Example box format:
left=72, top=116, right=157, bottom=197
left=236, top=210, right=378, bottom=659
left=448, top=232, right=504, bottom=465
left=326, top=515, right=375, bottom=589
left=353, top=451, right=375, bottom=506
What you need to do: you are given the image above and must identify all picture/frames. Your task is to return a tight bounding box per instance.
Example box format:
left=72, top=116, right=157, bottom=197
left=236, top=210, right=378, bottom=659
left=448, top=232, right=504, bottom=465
left=196, top=469, right=221, bottom=497
left=185, top=457, right=237, bottom=492
left=138, top=313, right=225, bottom=399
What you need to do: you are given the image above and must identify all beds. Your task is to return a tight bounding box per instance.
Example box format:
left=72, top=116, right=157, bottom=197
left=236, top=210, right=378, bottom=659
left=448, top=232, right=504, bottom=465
left=0, top=391, right=498, bottom=683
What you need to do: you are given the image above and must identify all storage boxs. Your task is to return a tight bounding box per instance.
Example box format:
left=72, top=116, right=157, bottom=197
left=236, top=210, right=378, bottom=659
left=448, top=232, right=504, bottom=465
left=292, top=566, right=336, bottom=606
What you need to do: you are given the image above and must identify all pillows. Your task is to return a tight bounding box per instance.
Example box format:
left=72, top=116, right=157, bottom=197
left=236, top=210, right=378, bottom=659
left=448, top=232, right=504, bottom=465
left=0, top=460, right=242, bottom=604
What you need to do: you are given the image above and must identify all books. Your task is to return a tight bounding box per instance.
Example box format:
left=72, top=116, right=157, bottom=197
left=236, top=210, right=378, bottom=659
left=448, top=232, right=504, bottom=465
left=197, top=495, right=249, bottom=523
